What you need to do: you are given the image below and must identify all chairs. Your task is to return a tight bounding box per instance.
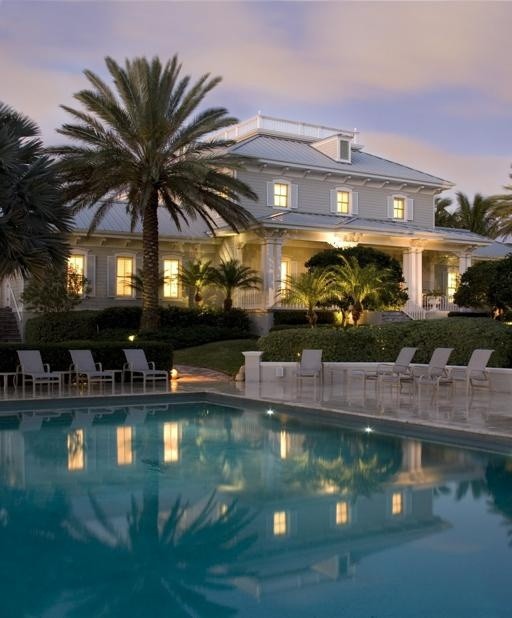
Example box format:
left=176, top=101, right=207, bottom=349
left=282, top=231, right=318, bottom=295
left=289, top=338, right=498, bottom=415
left=3, top=344, right=173, bottom=393
